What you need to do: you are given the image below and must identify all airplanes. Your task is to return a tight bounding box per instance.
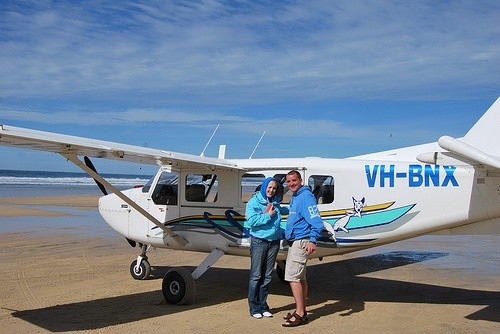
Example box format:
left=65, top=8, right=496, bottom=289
left=0, top=96, right=500, bottom=306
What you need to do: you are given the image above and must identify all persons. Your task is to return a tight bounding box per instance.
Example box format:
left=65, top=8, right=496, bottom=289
left=282, top=171, right=324, bottom=327
left=246, top=177, right=282, bottom=319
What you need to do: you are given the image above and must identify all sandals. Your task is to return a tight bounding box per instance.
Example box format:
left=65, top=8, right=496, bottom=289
left=283, top=310, right=307, bottom=319
left=282, top=313, right=308, bottom=327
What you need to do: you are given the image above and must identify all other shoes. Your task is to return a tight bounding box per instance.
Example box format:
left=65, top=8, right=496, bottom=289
left=263, top=312, right=272, bottom=317
left=252, top=313, right=262, bottom=319
